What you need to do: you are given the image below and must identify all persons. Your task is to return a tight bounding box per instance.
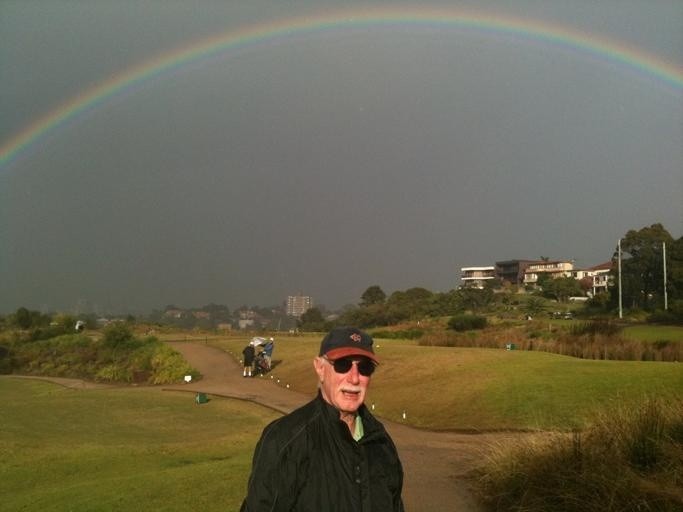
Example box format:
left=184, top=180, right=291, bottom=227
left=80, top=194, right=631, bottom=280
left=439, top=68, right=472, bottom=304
left=240, top=326, right=406, bottom=512
left=242, top=342, right=256, bottom=377
left=262, top=338, right=275, bottom=371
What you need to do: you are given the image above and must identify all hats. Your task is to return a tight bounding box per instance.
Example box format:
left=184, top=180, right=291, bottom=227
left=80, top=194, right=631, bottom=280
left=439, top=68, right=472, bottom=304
left=319, top=327, right=380, bottom=366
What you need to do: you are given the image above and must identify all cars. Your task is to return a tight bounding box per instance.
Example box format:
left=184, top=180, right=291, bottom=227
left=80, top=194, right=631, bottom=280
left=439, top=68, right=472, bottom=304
left=564, top=312, right=574, bottom=320
left=552, top=311, right=562, bottom=319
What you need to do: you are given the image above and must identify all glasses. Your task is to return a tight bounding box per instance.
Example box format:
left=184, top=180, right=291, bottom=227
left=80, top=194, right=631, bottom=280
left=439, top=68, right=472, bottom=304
left=322, top=356, right=375, bottom=375
